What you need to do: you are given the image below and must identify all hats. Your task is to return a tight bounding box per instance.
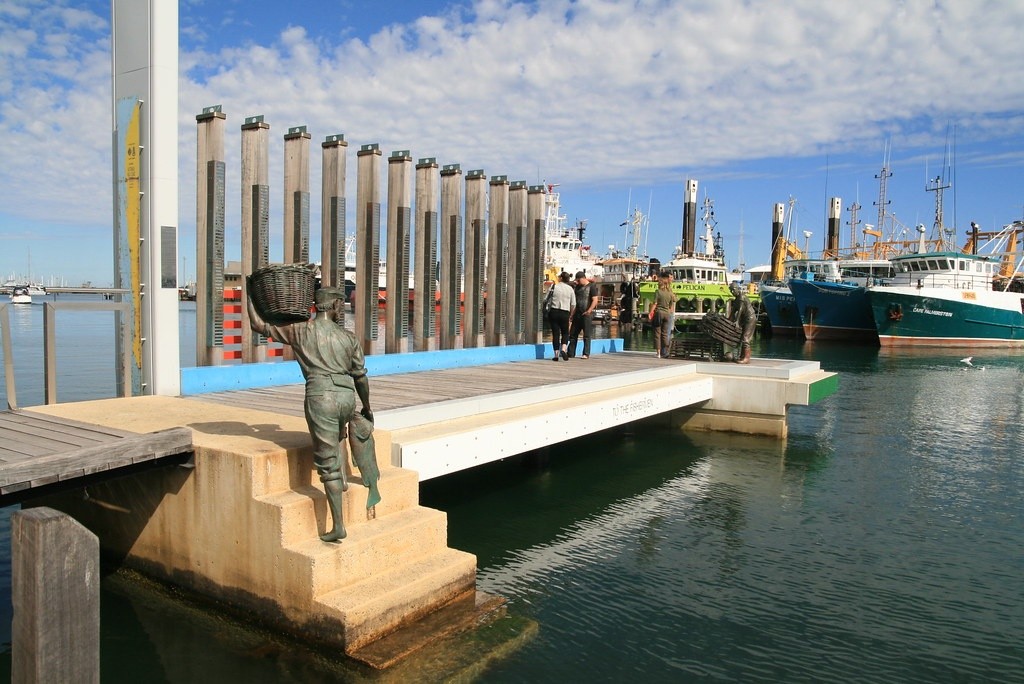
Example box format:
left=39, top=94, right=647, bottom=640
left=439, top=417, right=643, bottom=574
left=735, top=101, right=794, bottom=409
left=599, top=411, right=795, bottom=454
left=574, top=271, right=586, bottom=281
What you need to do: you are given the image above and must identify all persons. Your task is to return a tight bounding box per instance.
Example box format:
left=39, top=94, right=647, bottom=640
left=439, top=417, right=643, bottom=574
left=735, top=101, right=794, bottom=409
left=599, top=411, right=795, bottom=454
left=246, top=262, right=374, bottom=541
left=727, top=283, right=757, bottom=364
left=544, top=271, right=598, bottom=361
left=649, top=272, right=677, bottom=359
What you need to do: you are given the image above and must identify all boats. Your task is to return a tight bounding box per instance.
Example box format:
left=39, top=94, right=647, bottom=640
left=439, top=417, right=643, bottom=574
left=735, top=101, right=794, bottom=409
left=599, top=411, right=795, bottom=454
left=544, top=124, right=958, bottom=340
left=0, top=268, right=50, bottom=304
left=314, top=233, right=440, bottom=303
left=865, top=127, right=1024, bottom=347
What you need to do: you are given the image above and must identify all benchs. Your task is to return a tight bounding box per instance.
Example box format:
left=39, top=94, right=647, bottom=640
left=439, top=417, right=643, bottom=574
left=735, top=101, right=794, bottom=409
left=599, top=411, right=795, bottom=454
left=667, top=324, right=724, bottom=362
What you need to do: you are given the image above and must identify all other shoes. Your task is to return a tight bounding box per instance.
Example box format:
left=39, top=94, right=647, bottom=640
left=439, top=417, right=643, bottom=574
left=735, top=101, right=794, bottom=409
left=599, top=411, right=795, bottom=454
left=560, top=350, right=568, bottom=361
left=664, top=353, right=670, bottom=358
left=581, top=354, right=589, bottom=359
left=552, top=357, right=558, bottom=361
left=654, top=354, right=660, bottom=358
left=567, top=355, right=575, bottom=358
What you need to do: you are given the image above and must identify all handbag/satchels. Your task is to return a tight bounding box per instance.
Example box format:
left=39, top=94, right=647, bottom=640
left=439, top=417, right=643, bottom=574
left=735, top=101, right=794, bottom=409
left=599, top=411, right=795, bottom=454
left=652, top=311, right=662, bottom=327
left=543, top=284, right=555, bottom=312
